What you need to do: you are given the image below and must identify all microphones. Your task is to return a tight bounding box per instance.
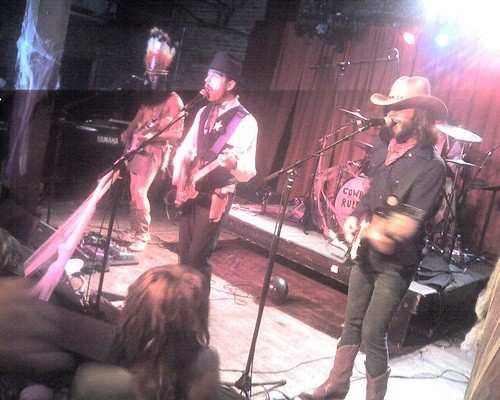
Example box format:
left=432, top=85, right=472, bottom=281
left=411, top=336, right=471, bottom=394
left=132, top=75, right=149, bottom=86
left=395, top=52, right=400, bottom=79
left=486, top=145, right=500, bottom=155
left=182, top=89, right=208, bottom=111
left=356, top=117, right=392, bottom=127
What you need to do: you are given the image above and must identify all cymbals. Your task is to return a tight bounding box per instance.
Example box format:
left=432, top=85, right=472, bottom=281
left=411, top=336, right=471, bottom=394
left=337, top=106, right=367, bottom=121
left=445, top=155, right=477, bottom=167
left=436, top=124, right=483, bottom=142
left=356, top=140, right=374, bottom=151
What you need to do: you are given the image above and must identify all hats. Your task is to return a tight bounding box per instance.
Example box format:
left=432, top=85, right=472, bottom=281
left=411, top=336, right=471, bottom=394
left=370, top=76, right=448, bottom=121
left=208, top=50, right=243, bottom=82
left=145, top=27, right=180, bottom=75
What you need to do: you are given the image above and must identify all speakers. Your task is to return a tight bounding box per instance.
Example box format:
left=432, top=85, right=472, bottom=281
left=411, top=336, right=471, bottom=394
left=0, top=184, right=83, bottom=313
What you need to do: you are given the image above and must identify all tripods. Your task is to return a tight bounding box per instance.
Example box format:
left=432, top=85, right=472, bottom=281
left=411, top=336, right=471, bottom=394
left=218, top=125, right=370, bottom=400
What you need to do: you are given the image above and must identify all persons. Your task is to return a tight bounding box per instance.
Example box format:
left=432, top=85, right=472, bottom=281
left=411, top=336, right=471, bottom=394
left=298, top=76, right=449, bottom=400
left=172, top=52, right=258, bottom=292
left=71, top=264, right=220, bottom=400
left=119, top=37, right=185, bottom=252
left=0, top=230, right=25, bottom=277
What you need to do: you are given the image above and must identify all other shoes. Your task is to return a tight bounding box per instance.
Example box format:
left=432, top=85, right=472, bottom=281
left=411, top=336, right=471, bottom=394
left=128, top=239, right=147, bottom=252
left=119, top=231, right=134, bottom=241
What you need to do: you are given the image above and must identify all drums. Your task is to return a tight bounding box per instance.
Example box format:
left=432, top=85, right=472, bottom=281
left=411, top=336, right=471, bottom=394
left=334, top=176, right=372, bottom=229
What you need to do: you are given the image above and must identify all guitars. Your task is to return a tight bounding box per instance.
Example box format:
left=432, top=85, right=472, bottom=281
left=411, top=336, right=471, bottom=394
left=174, top=154, right=240, bottom=209
left=121, top=117, right=158, bottom=153
left=341, top=204, right=423, bottom=268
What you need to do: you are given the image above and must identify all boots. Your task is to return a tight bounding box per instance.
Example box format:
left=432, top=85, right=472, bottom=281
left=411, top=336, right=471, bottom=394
left=364, top=361, right=391, bottom=400
left=298, top=337, right=360, bottom=400
